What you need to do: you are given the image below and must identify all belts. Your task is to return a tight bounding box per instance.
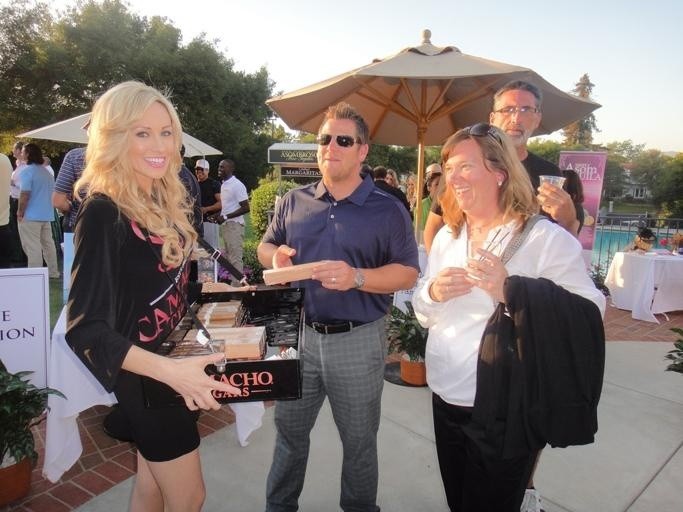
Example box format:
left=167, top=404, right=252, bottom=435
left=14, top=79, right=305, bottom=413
left=305, top=320, right=367, bottom=334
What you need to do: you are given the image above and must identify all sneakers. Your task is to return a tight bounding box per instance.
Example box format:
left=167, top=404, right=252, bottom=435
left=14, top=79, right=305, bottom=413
left=519, top=490, right=547, bottom=512
left=49, top=272, right=60, bottom=278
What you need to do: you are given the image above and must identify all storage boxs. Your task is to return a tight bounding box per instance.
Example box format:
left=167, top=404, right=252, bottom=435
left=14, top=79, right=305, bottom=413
left=144, top=286, right=308, bottom=409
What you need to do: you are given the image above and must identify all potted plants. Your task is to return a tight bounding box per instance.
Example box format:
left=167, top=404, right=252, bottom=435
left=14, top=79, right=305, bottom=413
left=0, top=359, right=68, bottom=507
left=385, top=298, right=430, bottom=387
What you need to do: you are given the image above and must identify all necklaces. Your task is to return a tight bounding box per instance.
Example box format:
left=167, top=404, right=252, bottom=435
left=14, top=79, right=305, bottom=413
left=469, top=207, right=503, bottom=232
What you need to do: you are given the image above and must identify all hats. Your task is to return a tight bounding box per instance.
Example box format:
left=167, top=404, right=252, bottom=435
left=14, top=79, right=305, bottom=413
left=194, top=159, right=209, bottom=170
left=82, top=118, right=91, bottom=129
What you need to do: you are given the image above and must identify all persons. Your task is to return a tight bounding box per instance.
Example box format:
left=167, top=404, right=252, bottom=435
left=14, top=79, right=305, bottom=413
left=258, top=103, right=421, bottom=512
left=360, top=162, right=418, bottom=221
left=217, top=159, right=251, bottom=287
left=175, top=141, right=201, bottom=236
left=192, top=158, right=221, bottom=252
left=489, top=80, right=584, bottom=236
left=412, top=122, right=607, bottom=510
left=50, top=147, right=110, bottom=304
left=559, top=167, right=584, bottom=232
left=0, top=141, right=62, bottom=279
left=64, top=81, right=259, bottom=511
left=412, top=161, right=443, bottom=232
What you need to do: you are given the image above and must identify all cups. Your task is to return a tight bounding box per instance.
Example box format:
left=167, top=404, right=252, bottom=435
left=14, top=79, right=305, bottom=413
left=538, top=174, right=565, bottom=190
left=466, top=239, right=500, bottom=278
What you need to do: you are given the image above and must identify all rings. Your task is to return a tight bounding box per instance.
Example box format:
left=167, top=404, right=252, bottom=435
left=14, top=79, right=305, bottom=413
left=331, top=276, right=337, bottom=285
left=480, top=271, right=488, bottom=286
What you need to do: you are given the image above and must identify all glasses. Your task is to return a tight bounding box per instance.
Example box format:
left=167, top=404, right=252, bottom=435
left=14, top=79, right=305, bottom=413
left=464, top=123, right=504, bottom=145
left=318, top=134, right=362, bottom=149
left=494, top=106, right=542, bottom=115
left=196, top=168, right=204, bottom=171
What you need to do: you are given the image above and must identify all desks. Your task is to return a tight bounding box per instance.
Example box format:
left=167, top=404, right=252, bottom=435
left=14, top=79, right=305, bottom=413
left=603, top=250, right=682, bottom=325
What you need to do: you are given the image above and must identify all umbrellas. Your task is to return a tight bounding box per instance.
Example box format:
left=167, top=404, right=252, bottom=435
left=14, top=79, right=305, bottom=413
left=17, top=107, right=224, bottom=157
left=264, top=30, right=602, bottom=225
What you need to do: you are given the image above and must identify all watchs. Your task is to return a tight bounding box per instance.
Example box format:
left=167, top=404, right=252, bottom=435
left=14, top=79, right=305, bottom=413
left=352, top=267, right=366, bottom=289
